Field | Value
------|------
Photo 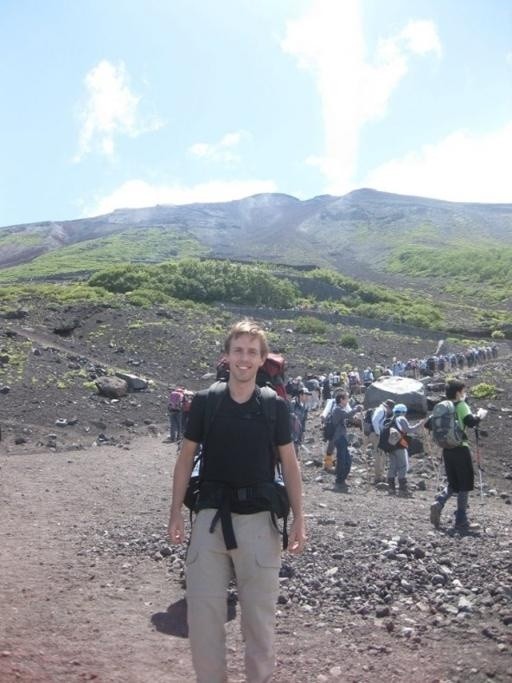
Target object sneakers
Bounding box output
[429,502,443,529]
[325,454,417,489]
[454,519,481,530]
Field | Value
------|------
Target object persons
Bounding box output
[365,399,426,491]
[322,387,358,484]
[167,387,194,442]
[289,387,312,439]
[168,321,306,682]
[428,378,482,531]
[284,343,498,411]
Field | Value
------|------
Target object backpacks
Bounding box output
[363,407,385,435]
[378,415,403,452]
[323,412,336,440]
[201,351,289,449]
[432,399,466,449]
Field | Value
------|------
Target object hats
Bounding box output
[297,388,313,396]
[382,399,407,413]
